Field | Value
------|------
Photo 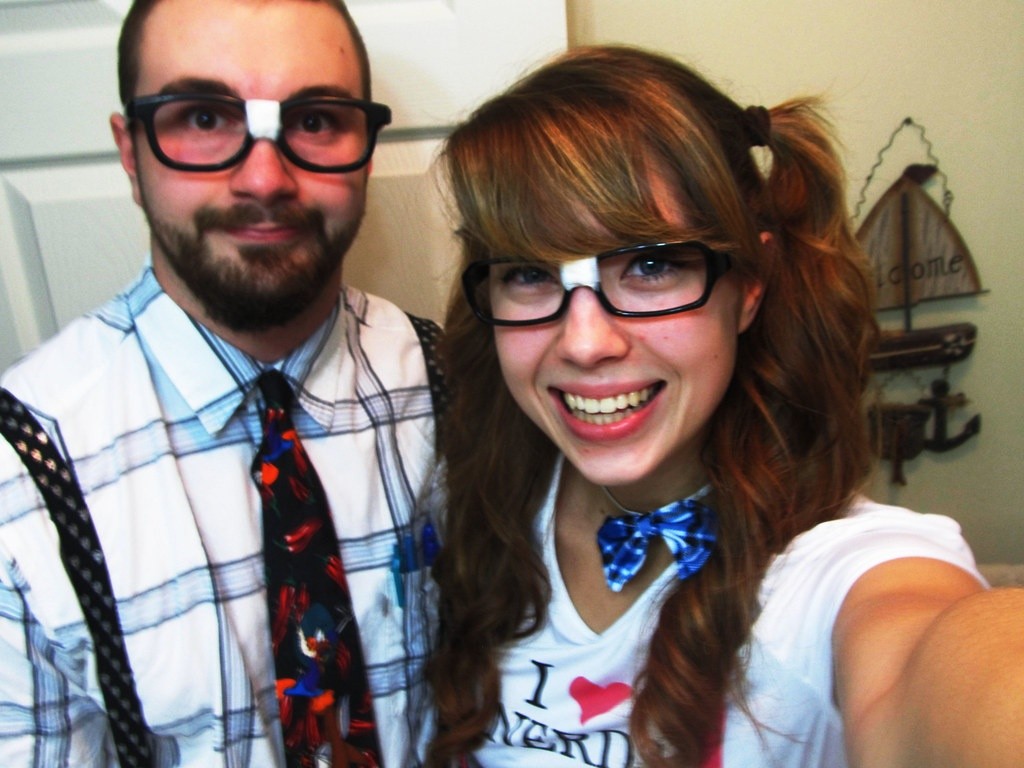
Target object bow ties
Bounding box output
[596,500,719,591]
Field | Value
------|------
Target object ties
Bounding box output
[250,372,383,768]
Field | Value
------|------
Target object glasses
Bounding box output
[127,92,391,174]
[461,241,734,327]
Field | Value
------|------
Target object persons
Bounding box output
[0,0,451,768]
[417,48,1024,768]
